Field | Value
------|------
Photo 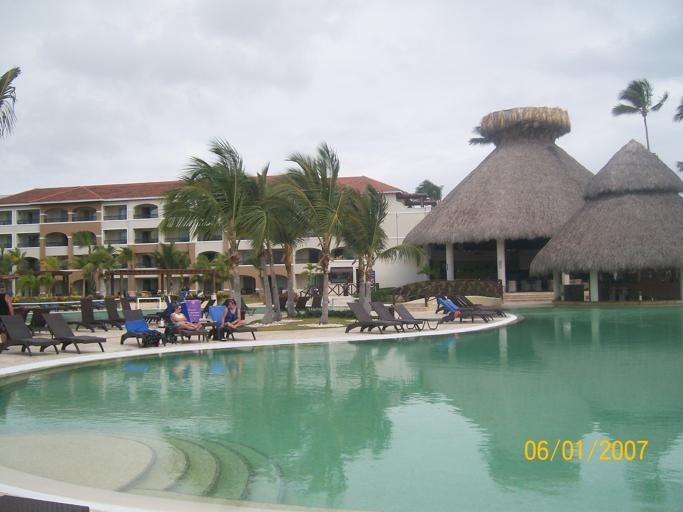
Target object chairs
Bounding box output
[271,293,323,315]
[344,301,443,334]
[0,293,107,357]
[436,294,506,324]
[68,293,260,349]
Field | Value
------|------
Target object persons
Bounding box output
[170,303,202,330]
[0,284,14,350]
[220,300,246,329]
